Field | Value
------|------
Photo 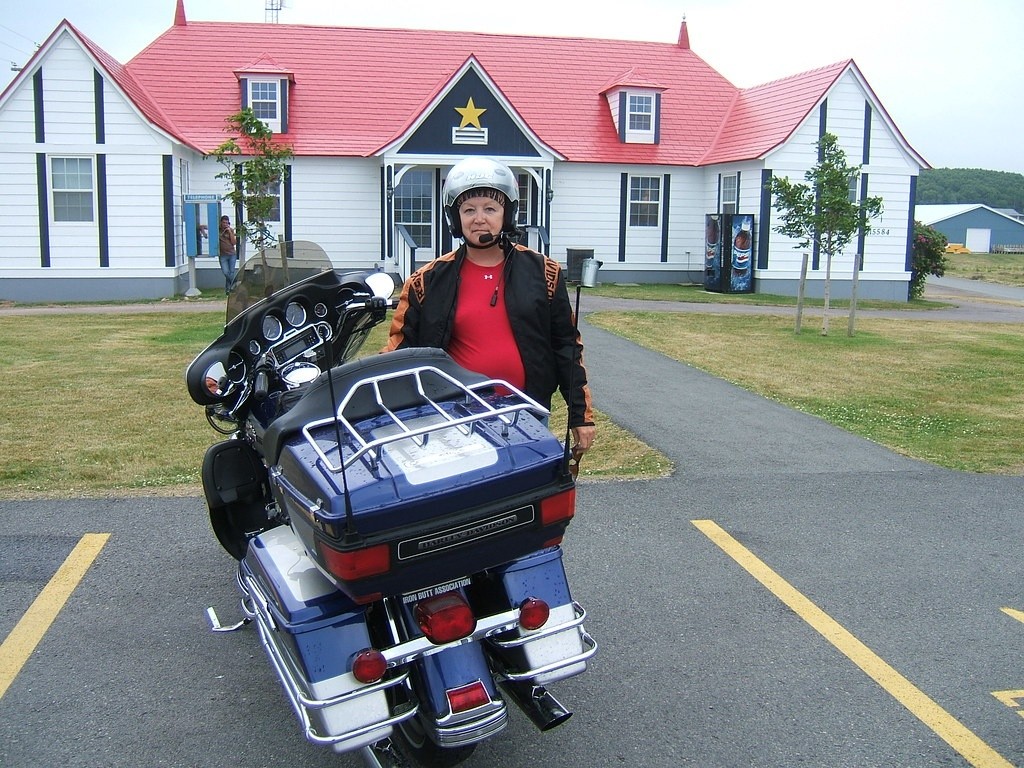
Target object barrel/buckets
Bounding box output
[566,248,595,282]
[384,261,394,273]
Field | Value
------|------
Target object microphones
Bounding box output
[479,231,515,243]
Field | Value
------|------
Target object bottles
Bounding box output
[374,262,378,272]
[705,215,720,269]
[732,221,752,276]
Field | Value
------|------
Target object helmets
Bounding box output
[442,156,526,239]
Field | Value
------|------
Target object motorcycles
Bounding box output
[186,242,598,768]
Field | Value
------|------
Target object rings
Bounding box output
[592,439,595,442]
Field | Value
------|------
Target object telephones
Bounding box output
[224,222,230,229]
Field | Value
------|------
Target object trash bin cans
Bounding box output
[581,257,603,289]
[566,248,595,283]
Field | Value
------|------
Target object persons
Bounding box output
[375,157,598,452]
[199,215,236,295]
